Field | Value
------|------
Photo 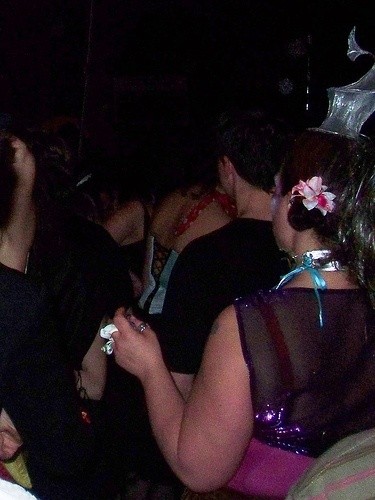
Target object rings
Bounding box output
[138,320,149,334]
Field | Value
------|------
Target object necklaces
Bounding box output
[282,248,347,275]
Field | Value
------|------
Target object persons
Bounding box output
[112,137,374,500]
[1,41,229,500]
[158,122,290,411]
